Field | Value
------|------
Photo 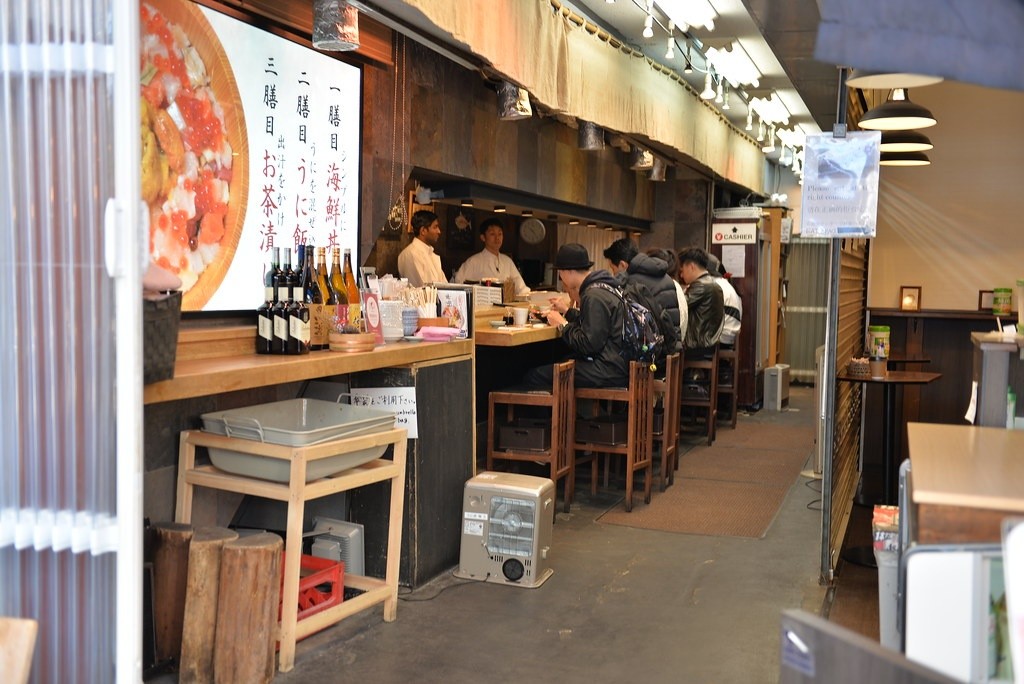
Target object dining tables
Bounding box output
[475,318,564,346]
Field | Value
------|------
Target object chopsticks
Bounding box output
[416,286,437,318]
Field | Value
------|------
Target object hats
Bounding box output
[706,254,723,277]
[548,243,595,269]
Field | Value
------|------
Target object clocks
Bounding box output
[519,219,546,245]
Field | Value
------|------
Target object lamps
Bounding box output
[845,67,944,88]
[606,0,803,186]
[881,130,934,152]
[880,152,931,166]
[858,88,938,130]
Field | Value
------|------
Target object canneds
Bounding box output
[993,287,1013,316]
[867,325,891,359]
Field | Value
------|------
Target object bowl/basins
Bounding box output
[403,308,418,336]
[490,321,506,327]
[533,312,564,323]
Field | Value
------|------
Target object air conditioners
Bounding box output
[453,471,554,589]
[764,364,791,413]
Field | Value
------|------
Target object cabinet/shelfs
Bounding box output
[712,202,793,416]
[780,421,1024,684]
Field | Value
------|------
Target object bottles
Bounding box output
[993,288,1012,315]
[870,326,890,356]
[256,244,361,354]
[502,307,514,326]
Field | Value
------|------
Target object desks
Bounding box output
[887,355,931,371]
[838,371,941,506]
[971,332,1024,429]
[144,324,477,593]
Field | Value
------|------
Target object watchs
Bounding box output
[557,321,568,333]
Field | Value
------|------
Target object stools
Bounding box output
[680,340,719,446]
[487,332,684,512]
[717,331,739,430]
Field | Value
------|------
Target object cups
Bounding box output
[418,318,449,330]
[513,308,528,325]
[869,361,887,377]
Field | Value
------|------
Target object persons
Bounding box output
[397,210,448,288]
[516,238,743,466]
[454,219,531,295]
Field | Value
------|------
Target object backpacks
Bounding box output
[585,282,665,372]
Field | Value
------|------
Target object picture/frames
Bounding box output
[898,285,922,312]
[978,290,994,311]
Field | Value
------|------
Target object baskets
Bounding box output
[144,289,182,387]
